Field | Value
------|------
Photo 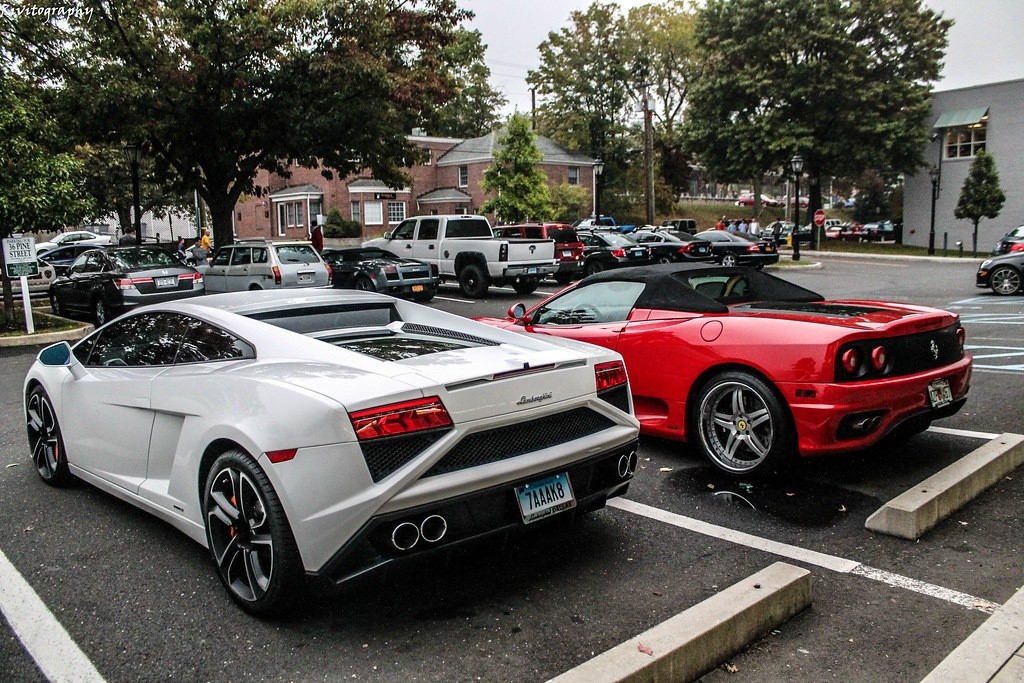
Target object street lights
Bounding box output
[927,163,942,254]
[125,138,150,245]
[592,155,610,229]
[791,150,805,259]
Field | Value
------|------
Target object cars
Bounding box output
[975,247,1024,297]
[691,228,781,274]
[624,224,712,267]
[573,228,653,277]
[991,224,1024,258]
[46,244,205,331]
[736,194,810,211]
[0,228,118,291]
[760,220,898,245]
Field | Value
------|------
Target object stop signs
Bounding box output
[813,210,828,228]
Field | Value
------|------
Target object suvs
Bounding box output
[489,220,587,287]
[189,238,333,299]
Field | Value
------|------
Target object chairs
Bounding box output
[694,281,728,300]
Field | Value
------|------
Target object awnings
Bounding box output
[933,106,988,128]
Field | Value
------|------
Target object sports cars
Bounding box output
[23,286,644,621]
[466,258,979,494]
[322,246,441,302]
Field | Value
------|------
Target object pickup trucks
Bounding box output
[357,214,560,297]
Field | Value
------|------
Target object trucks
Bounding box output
[574,216,640,234]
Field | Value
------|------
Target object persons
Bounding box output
[310,220,324,255]
[770,218,784,250]
[178,230,215,264]
[715,215,761,238]
[118,227,135,248]
